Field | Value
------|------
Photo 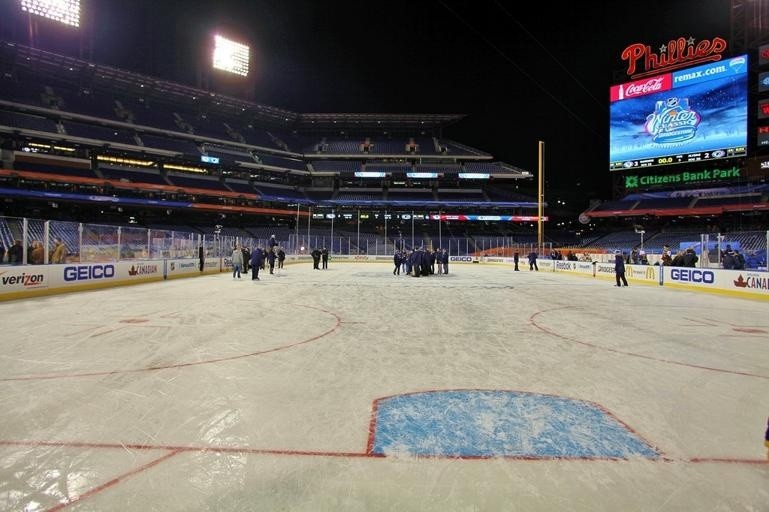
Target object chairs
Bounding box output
[552,190,769,273]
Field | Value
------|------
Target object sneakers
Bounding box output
[411,272,449,277]
[613,284,629,287]
[393,272,400,275]
[514,269,520,271]
[405,272,410,275]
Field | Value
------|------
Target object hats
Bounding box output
[613,250,621,254]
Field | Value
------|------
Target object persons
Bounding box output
[527,250,533,271]
[513,249,521,272]
[311,247,322,269]
[528,250,539,272]
[321,245,329,270]
[0,236,67,266]
[231,244,285,282]
[623,244,746,271]
[612,249,629,288]
[549,248,579,261]
[267,234,279,249]
[392,247,449,278]
